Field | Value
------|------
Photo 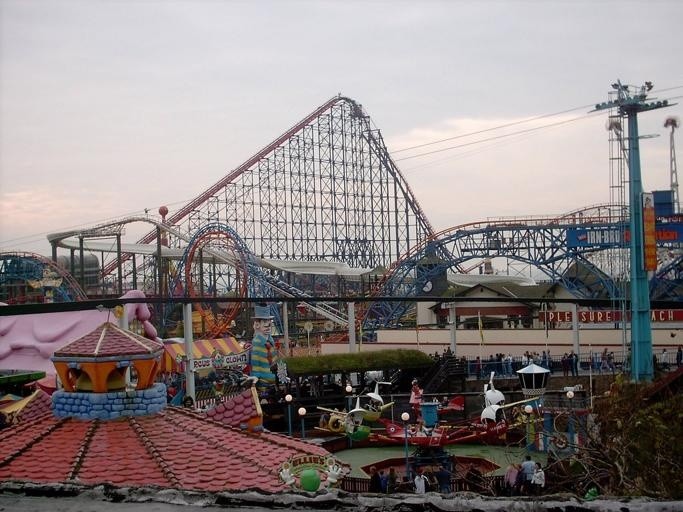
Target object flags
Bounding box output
[478,310,485,347]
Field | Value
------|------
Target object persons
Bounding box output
[514,455,545,493]
[429,347,466,369]
[415,467,451,494]
[653,347,683,371]
[432,396,451,407]
[600,347,631,371]
[476,353,513,379]
[513,405,527,422]
[562,350,579,375]
[522,349,554,374]
[370,399,380,412]
[240,306,279,393]
[371,466,398,494]
[466,464,481,493]
